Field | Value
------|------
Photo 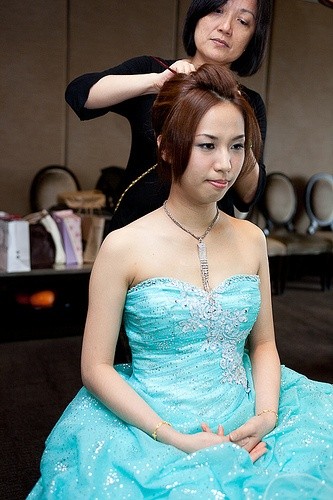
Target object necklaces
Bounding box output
[163,200,218,292]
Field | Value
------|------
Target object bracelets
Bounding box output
[151,421,171,440]
[255,409,278,423]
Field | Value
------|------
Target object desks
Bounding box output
[1,234,290,350]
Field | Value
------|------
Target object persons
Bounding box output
[24,63,332,500]
[64,0,269,234]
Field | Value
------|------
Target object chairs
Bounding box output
[305,172,333,291]
[250,205,289,295]
[258,172,329,295]
[30,165,81,213]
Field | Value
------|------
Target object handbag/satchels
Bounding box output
[0,190,105,273]
